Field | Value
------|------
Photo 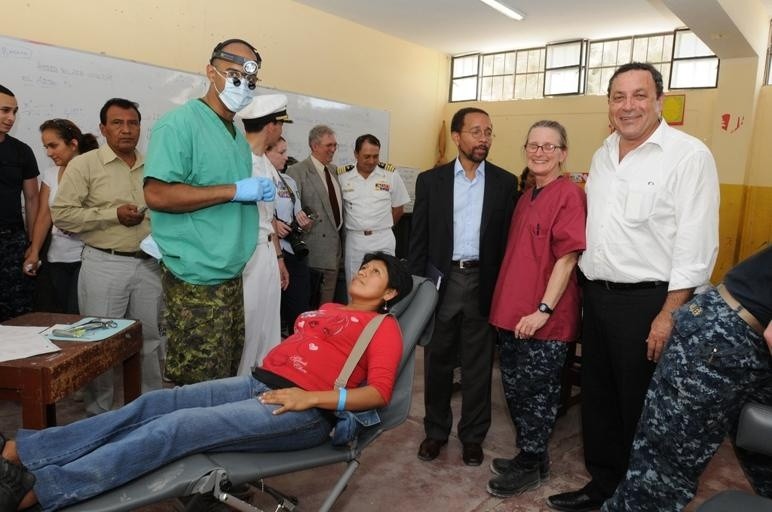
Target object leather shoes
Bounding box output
[461,442,484,468]
[545,477,620,512]
[417,433,449,462]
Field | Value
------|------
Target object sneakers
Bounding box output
[0,456,41,512]
[0,429,9,457]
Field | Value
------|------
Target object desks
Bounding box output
[2,312,144,431]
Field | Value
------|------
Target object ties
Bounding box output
[324,165,341,230]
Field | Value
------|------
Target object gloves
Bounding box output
[256,176,277,203]
[230,176,264,205]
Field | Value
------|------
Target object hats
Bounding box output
[235,93,296,126]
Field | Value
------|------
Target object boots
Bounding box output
[489,447,551,483]
[485,450,542,499]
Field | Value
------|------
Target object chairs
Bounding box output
[44,272,441,510]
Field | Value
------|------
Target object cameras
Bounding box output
[285,206,313,261]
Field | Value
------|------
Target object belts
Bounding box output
[586,278,670,293]
[346,227,392,236]
[449,259,480,269]
[86,241,155,261]
[715,282,765,337]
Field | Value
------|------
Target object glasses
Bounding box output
[523,141,565,154]
[456,129,496,139]
[211,62,261,86]
[316,142,340,150]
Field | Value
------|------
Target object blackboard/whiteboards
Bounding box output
[0,35,393,214]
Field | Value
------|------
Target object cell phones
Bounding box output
[139,207,148,213]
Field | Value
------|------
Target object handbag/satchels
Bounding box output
[329,409,382,446]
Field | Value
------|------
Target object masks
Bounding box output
[212,65,257,113]
